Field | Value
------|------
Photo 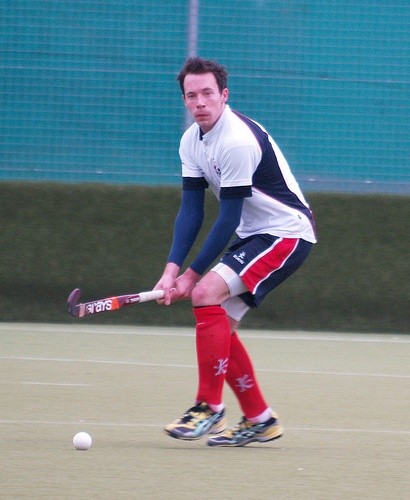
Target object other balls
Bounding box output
[73,432,92,450]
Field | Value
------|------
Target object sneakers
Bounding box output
[164,401,226,441]
[206,410,284,448]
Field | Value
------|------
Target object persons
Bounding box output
[154,56,318,447]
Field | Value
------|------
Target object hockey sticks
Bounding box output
[67,287,175,319]
